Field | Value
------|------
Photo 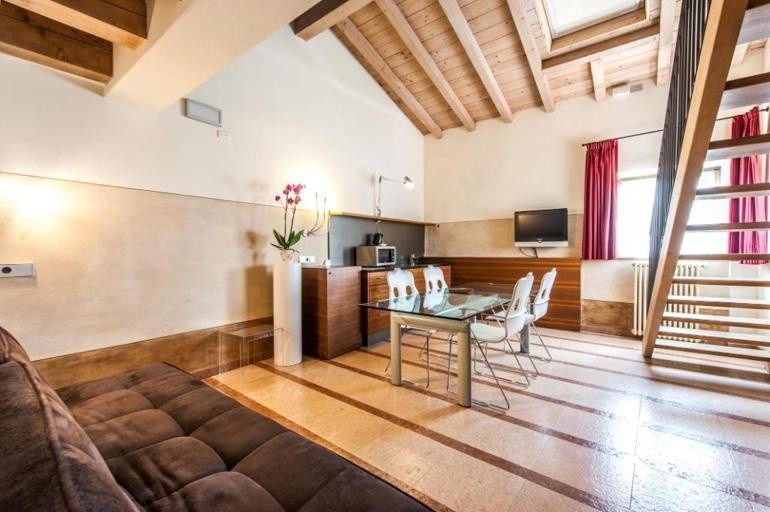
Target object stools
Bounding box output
[217,319,287,394]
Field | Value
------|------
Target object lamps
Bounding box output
[376,174,414,223]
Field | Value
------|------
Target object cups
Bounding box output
[322,260,331,267]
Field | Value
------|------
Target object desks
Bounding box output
[358,280,541,407]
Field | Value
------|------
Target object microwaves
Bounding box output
[354,246,397,268]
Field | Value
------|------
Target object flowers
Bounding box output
[269,183,306,250]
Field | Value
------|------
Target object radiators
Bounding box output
[629,261,706,343]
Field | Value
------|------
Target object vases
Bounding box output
[272,252,304,367]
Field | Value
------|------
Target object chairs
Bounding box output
[385,265,558,409]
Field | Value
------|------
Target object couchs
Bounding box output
[0,326,434,512]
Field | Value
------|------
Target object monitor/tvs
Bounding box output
[514,208,569,248]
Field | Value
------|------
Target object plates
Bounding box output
[449,287,475,294]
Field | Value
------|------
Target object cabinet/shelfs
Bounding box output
[302,263,363,360]
[361,264,452,347]
